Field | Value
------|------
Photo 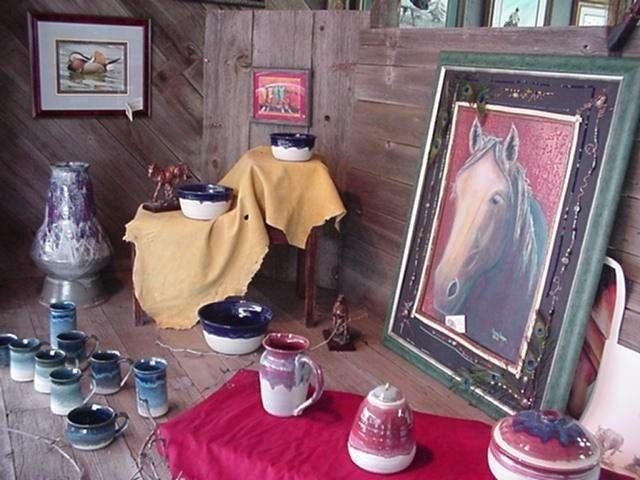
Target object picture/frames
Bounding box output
[250,67,314,129]
[378,48,638,423]
[26,9,153,119]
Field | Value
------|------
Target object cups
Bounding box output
[0,301,169,451]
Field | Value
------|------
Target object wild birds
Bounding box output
[66,50,121,75]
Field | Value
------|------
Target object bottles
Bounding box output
[347,383,417,474]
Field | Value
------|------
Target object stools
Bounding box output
[131,145,324,330]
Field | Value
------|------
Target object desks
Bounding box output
[168,368,637,480]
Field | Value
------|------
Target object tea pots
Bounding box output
[259,332,325,418]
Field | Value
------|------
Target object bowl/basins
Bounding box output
[198,301,275,356]
[178,182,234,220]
[269,132,316,162]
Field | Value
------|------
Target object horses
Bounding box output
[432,115,550,363]
